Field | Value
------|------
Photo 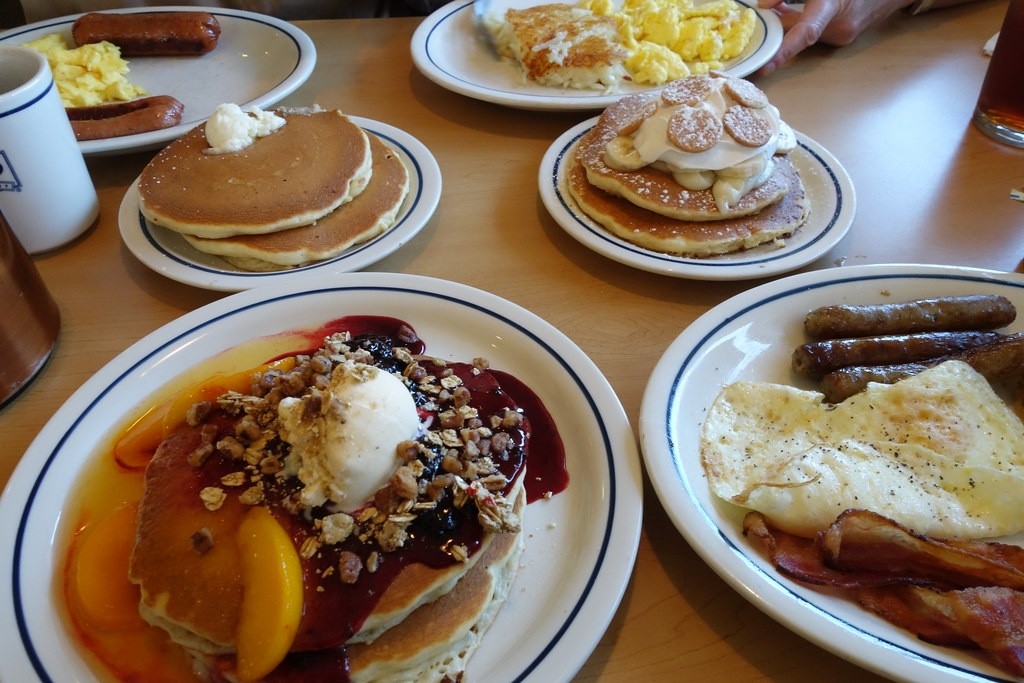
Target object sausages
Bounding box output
[63,95,183,138]
[72,11,221,57]
[793,292,1022,404]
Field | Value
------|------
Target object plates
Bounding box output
[536,112,858,283]
[115,110,442,294]
[639,264,1023,682]
[0,4,316,159]
[1,272,647,682]
[410,0,785,110]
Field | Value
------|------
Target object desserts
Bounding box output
[569,73,808,251]
[132,331,523,682]
[136,103,408,272]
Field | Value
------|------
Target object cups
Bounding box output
[0,46,100,255]
[2,212,65,411]
[970,1,1024,148]
